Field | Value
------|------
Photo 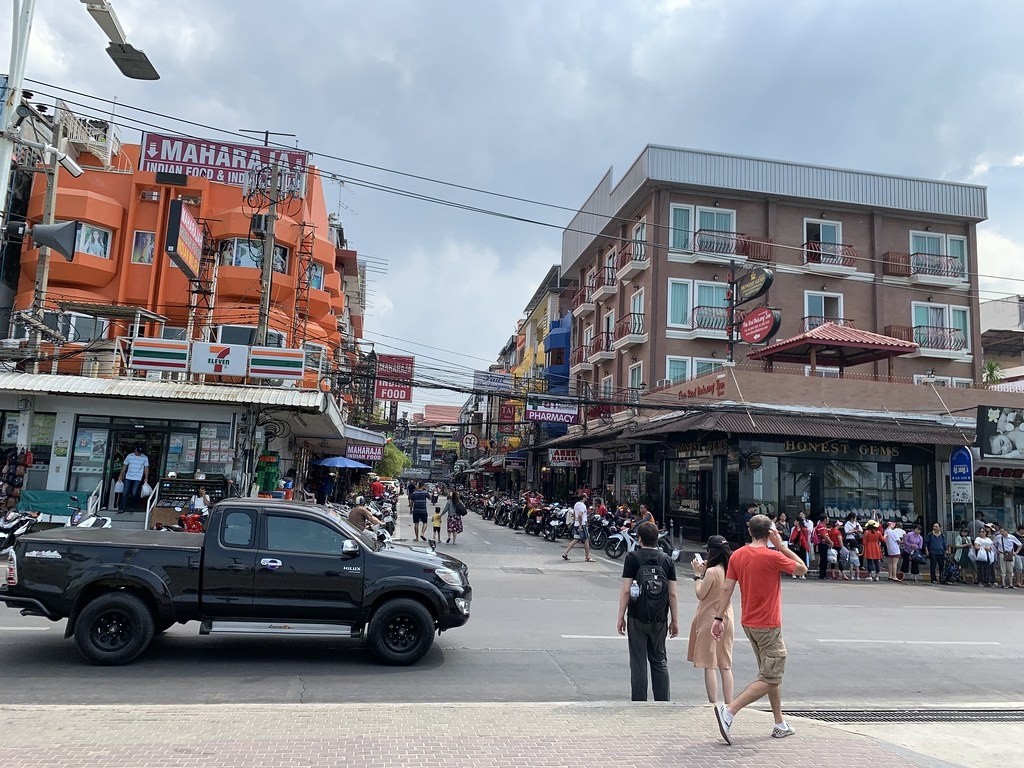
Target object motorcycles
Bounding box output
[432,490,439,505]
[0,505,41,552]
[153,506,208,534]
[322,486,398,550]
[457,486,673,559]
[64,496,113,529]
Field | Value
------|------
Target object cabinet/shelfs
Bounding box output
[157,478,225,506]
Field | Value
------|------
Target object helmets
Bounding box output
[355,495,365,505]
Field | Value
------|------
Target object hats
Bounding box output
[865,520,880,528]
[136,445,142,453]
[984,523,997,531]
[579,493,590,498]
[702,535,729,550]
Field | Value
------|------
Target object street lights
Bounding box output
[0,1,161,226]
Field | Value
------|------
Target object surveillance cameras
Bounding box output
[57,152,84,178]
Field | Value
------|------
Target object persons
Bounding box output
[688,535,734,703]
[617,521,680,701]
[311,263,320,290]
[767,510,1024,589]
[561,493,596,562]
[743,504,758,545]
[452,484,496,504]
[980,408,1024,459]
[431,507,442,543]
[191,486,210,525]
[710,515,808,746]
[438,492,465,544]
[117,446,149,514]
[321,472,385,502]
[410,482,431,541]
[349,496,386,540]
[222,241,285,272]
[83,229,104,257]
[401,480,451,506]
[595,498,655,528]
[519,489,543,528]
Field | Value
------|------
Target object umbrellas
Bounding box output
[309,455,373,470]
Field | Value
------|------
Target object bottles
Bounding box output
[7,555,16,585]
[630,580,639,602]
[58,437,67,447]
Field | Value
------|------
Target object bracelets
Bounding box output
[693,576,702,581]
[714,616,723,621]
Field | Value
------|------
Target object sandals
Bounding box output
[561,552,596,562]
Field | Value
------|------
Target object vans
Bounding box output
[379,479,401,495]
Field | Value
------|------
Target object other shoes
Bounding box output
[118,509,123,513]
[790,572,1024,590]
[413,534,457,545]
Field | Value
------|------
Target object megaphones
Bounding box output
[7,218,78,262]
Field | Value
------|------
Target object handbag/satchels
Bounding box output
[909,548,928,566]
[456,501,467,516]
[114,479,152,498]
[828,547,838,564]
[0,447,33,508]
[968,544,1013,561]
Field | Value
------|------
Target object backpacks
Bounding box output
[631,549,669,625]
[780,522,790,541]
[812,527,826,545]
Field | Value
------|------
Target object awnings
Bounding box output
[463,455,503,473]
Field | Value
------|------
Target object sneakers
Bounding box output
[714,704,795,745]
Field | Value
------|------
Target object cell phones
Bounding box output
[695,553,703,566]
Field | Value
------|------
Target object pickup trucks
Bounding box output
[1,497,472,667]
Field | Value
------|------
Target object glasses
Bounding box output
[753,508,756,511]
[979,530,985,533]
[200,487,205,490]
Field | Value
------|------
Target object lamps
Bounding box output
[631,356,637,364]
[604,306,611,311]
[640,380,647,389]
[927,294,933,302]
[715,200,720,207]
[821,212,826,218]
[821,283,828,290]
[587,264,593,271]
[928,367,937,379]
[633,284,640,291]
[714,273,719,281]
[711,349,717,358]
[586,320,591,326]
[635,214,642,222]
[604,369,609,375]
[608,242,613,250]
